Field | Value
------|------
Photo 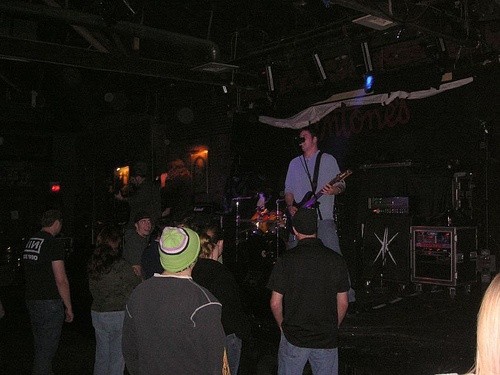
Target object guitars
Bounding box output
[287,168,353,235]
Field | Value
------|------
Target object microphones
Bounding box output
[294,136,305,143]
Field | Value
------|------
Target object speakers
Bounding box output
[361,214,423,285]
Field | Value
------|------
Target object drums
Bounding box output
[232,196,257,219]
[252,205,287,233]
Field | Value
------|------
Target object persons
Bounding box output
[23,166,349,375]
[284,126,355,302]
[461,273,500,375]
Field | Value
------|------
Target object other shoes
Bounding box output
[345,302,357,319]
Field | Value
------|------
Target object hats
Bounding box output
[158,226,200,272]
[294,208,317,233]
[134,212,151,223]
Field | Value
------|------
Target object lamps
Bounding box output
[311,54,327,82]
[359,42,376,93]
[435,37,448,57]
[265,65,279,97]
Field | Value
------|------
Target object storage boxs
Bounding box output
[408,226,479,287]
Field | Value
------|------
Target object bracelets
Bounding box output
[337,187,343,194]
